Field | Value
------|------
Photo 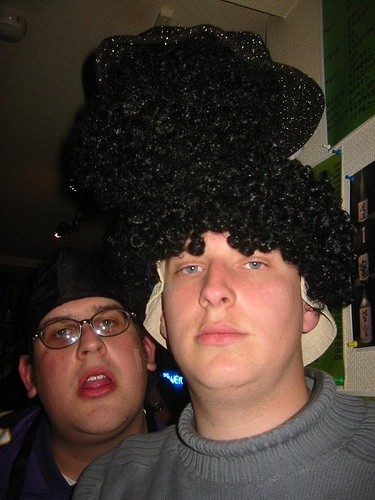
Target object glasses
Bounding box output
[30,307,142,350]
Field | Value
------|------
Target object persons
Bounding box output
[72,159,375,499]
[145,377,184,429]
[0,255,182,500]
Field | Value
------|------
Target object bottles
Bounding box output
[359,286,372,344]
[358,226,370,283]
[357,173,369,223]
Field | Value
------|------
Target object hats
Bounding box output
[24,246,129,355]
